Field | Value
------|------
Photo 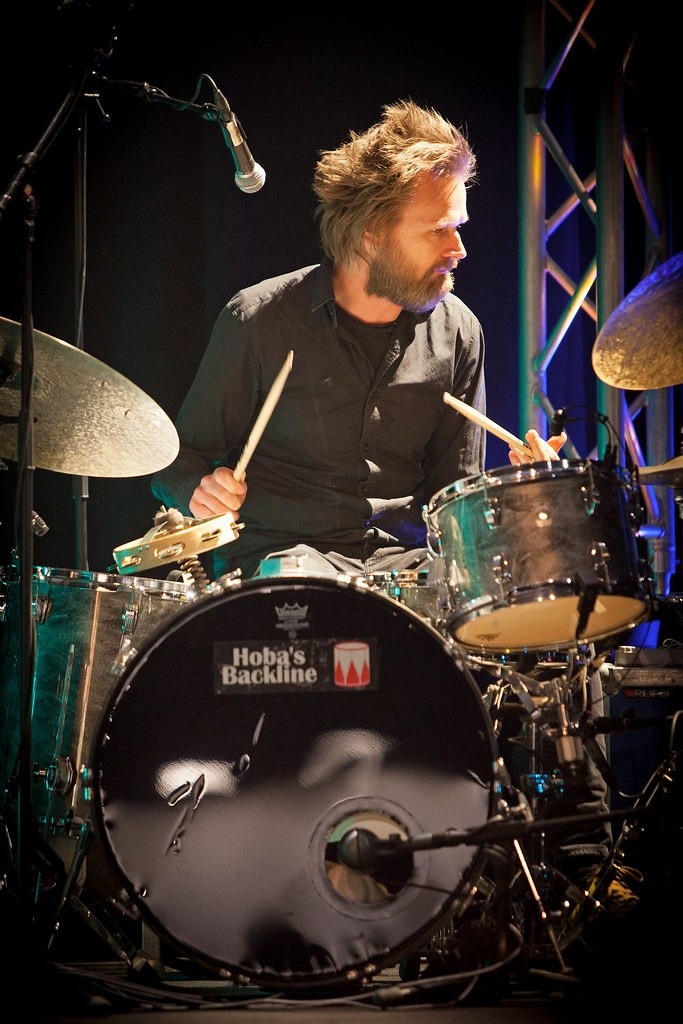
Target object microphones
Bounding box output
[213,89,266,193]
[626,715,674,730]
[549,409,566,440]
[337,828,476,871]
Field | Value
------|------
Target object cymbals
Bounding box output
[156,542,185,560]
[107,556,141,572]
[632,455,683,486]
[0,314,181,479]
[230,522,245,531]
[200,532,222,544]
[590,252,683,391]
[104,510,247,576]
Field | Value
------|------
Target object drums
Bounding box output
[30,564,224,838]
[87,576,498,985]
[427,458,651,653]
[338,571,442,631]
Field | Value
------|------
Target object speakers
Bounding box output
[590,665,683,901]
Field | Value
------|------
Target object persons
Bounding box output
[151,103,663,950]
